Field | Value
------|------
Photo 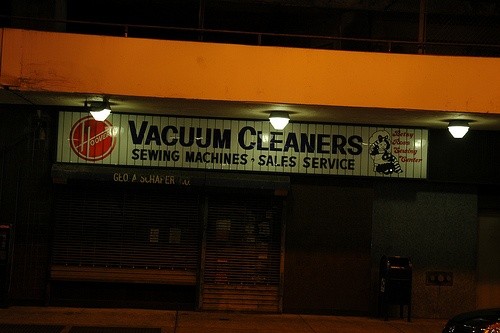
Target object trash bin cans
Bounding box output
[376,253,411,321]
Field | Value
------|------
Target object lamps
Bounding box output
[269,112,290,130]
[88,100,113,121]
[447,120,470,138]
